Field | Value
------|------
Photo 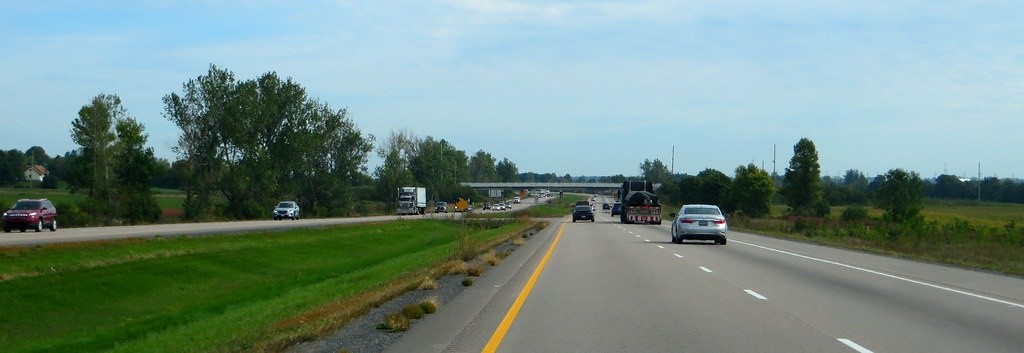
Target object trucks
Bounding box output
[396,186,426,215]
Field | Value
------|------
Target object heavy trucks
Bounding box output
[617,177,662,225]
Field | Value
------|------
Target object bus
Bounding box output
[456,197,474,211]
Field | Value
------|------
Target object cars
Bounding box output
[571,207,595,222]
[603,203,611,210]
[504,201,511,209]
[434,202,448,213]
[483,202,491,210]
[669,204,728,246]
[594,194,598,197]
[3,197,57,232]
[572,200,594,210]
[273,201,300,221]
[499,203,505,210]
[591,197,596,201]
[524,190,554,200]
[590,203,596,211]
[492,204,500,211]
[513,197,520,204]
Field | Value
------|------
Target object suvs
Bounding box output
[611,202,621,217]
